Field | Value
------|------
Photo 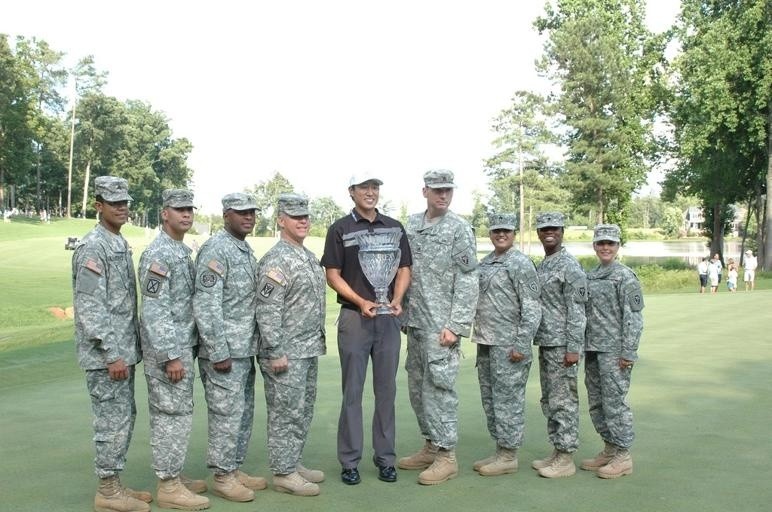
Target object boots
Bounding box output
[157,476,210,511]
[472,448,498,471]
[114,475,153,504]
[232,469,267,490]
[532,450,561,469]
[213,471,255,502]
[156,474,208,493]
[539,452,576,479]
[480,447,517,475]
[596,449,633,479]
[399,439,440,470]
[95,476,151,512]
[296,463,325,483]
[418,451,458,485]
[581,443,618,471]
[273,471,320,496]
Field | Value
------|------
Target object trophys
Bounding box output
[356,233,402,316]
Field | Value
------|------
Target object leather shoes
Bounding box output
[342,467,361,484]
[378,465,398,482]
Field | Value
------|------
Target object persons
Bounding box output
[74,175,153,512]
[139,190,212,511]
[192,192,267,503]
[726,265,738,294]
[533,210,589,481]
[698,258,708,294]
[254,193,328,498]
[397,170,479,487]
[727,258,738,292]
[708,258,719,294]
[742,250,758,292]
[712,254,722,293]
[584,224,644,481]
[322,166,411,485]
[474,212,541,477]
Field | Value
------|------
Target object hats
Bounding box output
[278,195,312,216]
[348,168,384,188]
[710,259,716,263]
[95,176,134,202]
[162,189,197,210]
[745,250,752,254]
[423,168,458,189]
[593,224,620,243]
[535,213,565,229]
[489,214,517,231]
[221,193,262,212]
